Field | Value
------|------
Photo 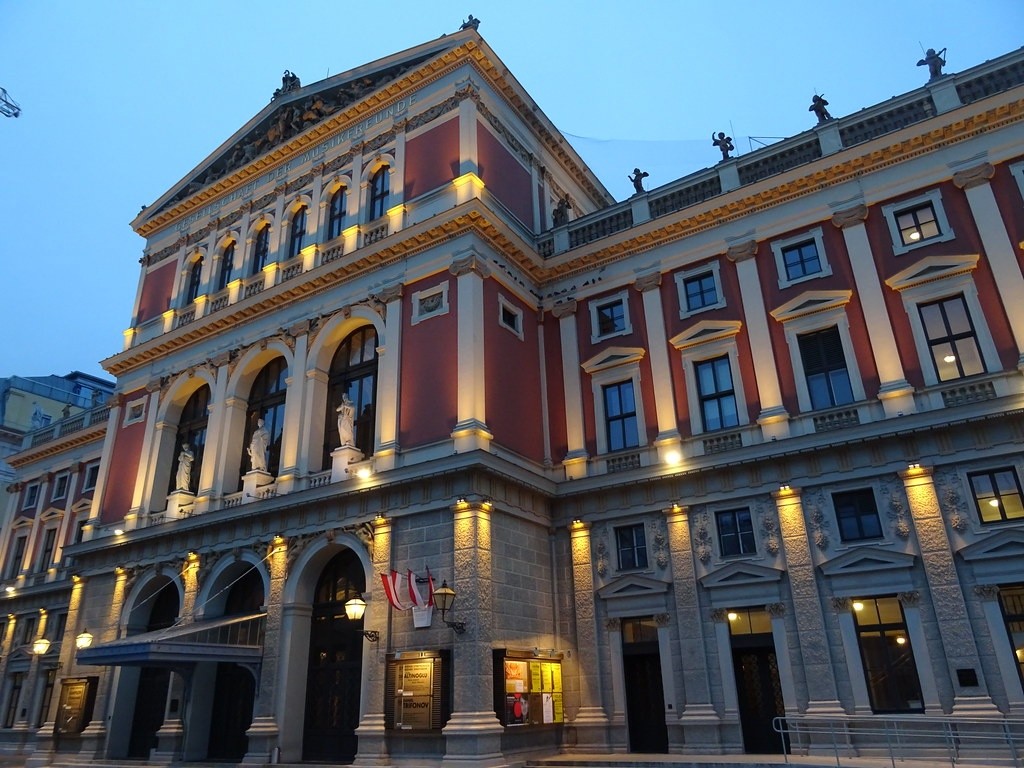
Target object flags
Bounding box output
[381,570,435,611]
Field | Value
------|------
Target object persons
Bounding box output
[553,198,572,225]
[280,70,301,94]
[809,95,833,122]
[628,168,649,193]
[337,393,355,447]
[176,443,194,491]
[460,14,481,31]
[925,48,948,76]
[711,132,734,159]
[61,401,73,418]
[91,388,103,406]
[247,418,269,471]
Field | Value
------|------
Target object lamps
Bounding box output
[76,628,94,647]
[33,634,51,654]
[344,579,466,642]
